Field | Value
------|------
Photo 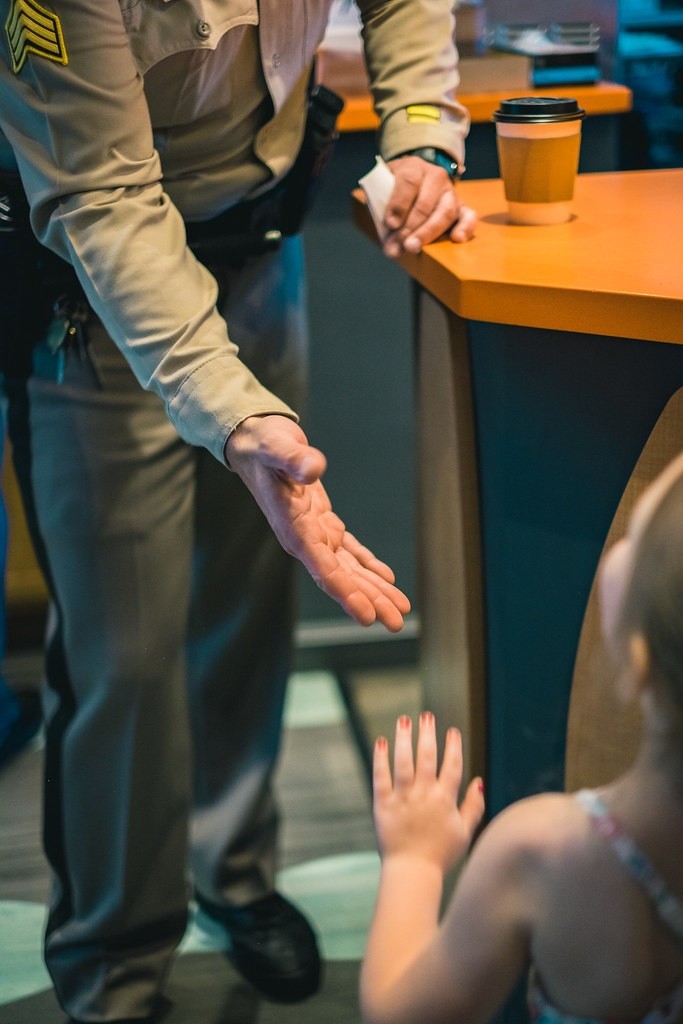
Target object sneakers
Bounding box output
[192,887,321,1002]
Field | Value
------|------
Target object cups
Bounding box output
[490,96,586,226]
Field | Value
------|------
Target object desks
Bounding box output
[348,163,683,1024]
[274,52,629,676]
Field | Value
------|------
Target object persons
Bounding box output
[358,455,682,1024]
[0,0,477,1022]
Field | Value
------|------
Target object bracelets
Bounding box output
[411,147,457,179]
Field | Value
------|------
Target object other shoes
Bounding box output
[0,688,43,762]
